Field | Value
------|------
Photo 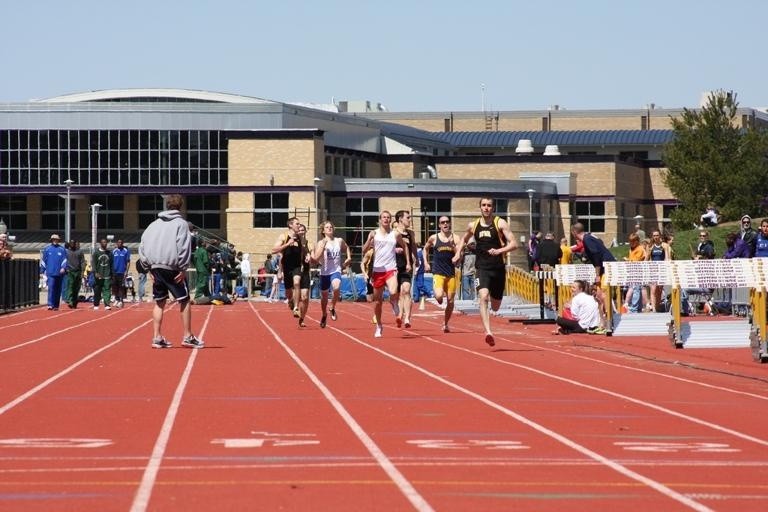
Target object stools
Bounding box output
[687,293,720,319]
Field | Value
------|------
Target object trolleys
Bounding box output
[682,287,723,318]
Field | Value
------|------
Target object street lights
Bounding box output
[0,218,9,235]
[63,178,75,242]
[268,174,276,228]
[311,175,323,244]
[632,213,643,228]
[525,188,537,236]
[90,202,102,252]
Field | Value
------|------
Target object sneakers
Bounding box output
[371,296,449,338]
[90,300,123,310]
[151,336,174,348]
[181,336,204,349]
[485,333,495,347]
[288,299,337,328]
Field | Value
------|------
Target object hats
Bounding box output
[49,234,61,241]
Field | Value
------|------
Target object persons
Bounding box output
[736,213,759,257]
[526,205,718,335]
[0,230,14,260]
[184,235,252,304]
[137,194,207,350]
[360,210,425,337]
[42,234,131,311]
[752,219,768,259]
[135,258,148,303]
[422,216,464,331]
[721,232,752,258]
[451,196,521,347]
[259,218,352,328]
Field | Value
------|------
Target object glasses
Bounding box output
[742,222,750,224]
[699,235,705,237]
